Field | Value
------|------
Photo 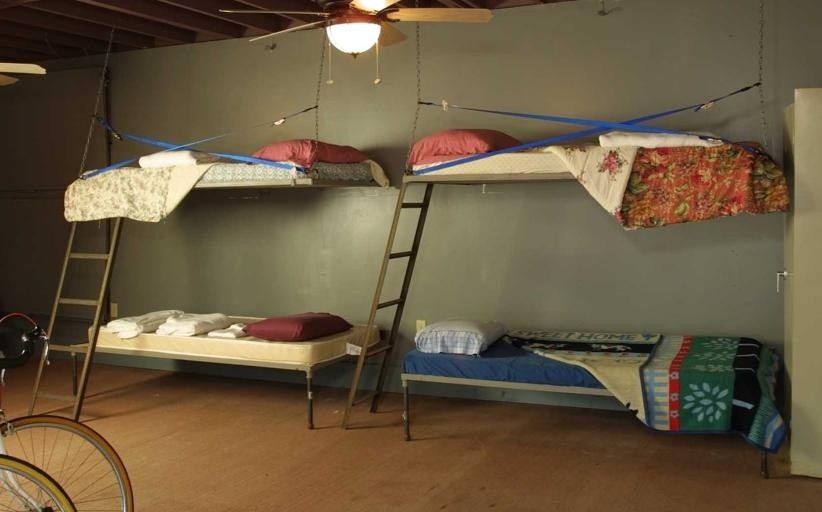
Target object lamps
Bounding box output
[323,16,381,86]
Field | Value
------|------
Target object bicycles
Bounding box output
[0,312,135,512]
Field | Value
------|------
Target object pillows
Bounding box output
[249,136,369,168]
[403,127,527,170]
[413,317,508,354]
[241,312,354,342]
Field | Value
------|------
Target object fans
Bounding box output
[217,1,496,42]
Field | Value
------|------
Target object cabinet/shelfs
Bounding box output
[775,86,822,480]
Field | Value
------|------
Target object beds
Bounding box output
[405,144,780,187]
[86,311,388,430]
[64,157,393,224]
[400,328,782,479]
